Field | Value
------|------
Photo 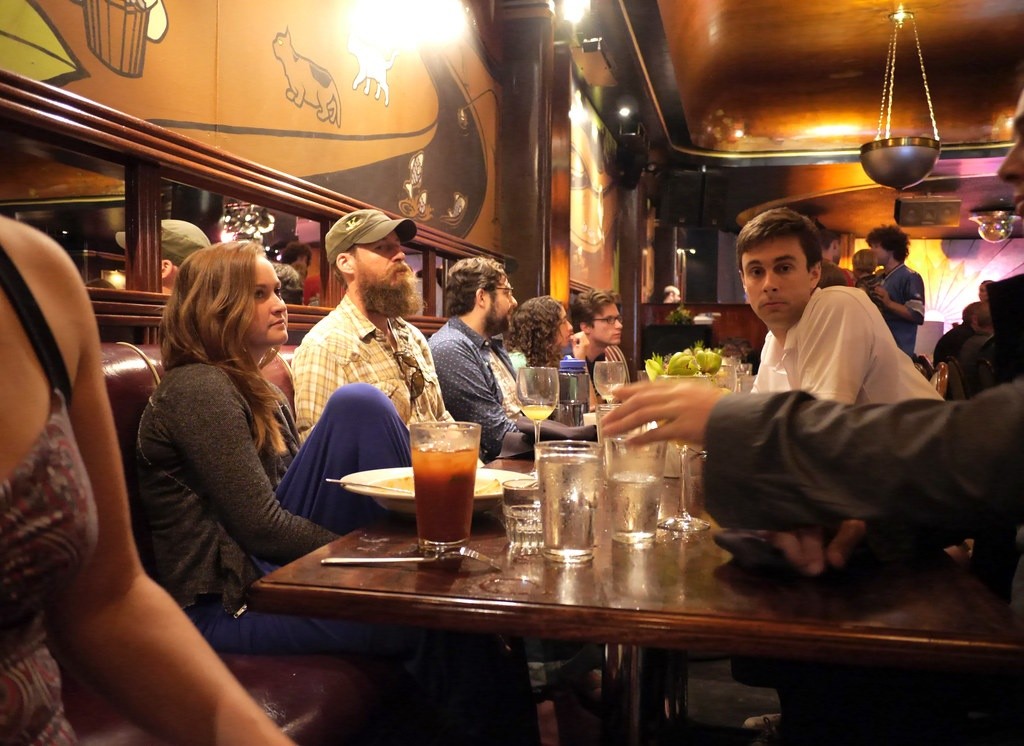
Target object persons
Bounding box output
[601,66,1024,746]
[115,218,213,294]
[131,211,635,745]
[0,213,296,746]
[815,224,925,361]
[735,205,980,746]
[932,280,994,392]
[266,261,304,306]
[662,285,681,303]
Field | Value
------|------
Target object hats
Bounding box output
[116,220,211,267]
[325,210,416,264]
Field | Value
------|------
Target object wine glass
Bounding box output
[656,417,711,533]
[515,365,560,477]
[593,361,626,404]
[714,364,738,393]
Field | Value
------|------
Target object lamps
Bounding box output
[969,211,1021,243]
[859,2,941,194]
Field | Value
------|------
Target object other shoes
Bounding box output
[741,712,782,746]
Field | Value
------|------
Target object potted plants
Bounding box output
[640,299,714,369]
[644,340,731,449]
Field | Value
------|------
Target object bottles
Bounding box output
[558,355,590,427]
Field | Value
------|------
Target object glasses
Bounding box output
[497,287,513,295]
[394,351,425,400]
[593,316,623,323]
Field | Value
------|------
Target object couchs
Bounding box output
[100,343,391,746]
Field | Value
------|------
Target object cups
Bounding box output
[595,403,640,442]
[604,434,667,545]
[535,439,603,566]
[501,478,544,550]
[409,421,482,558]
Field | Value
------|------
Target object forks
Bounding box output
[321,545,502,570]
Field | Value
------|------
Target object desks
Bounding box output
[246,459,1024,746]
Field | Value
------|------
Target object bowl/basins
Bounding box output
[341,467,533,516]
